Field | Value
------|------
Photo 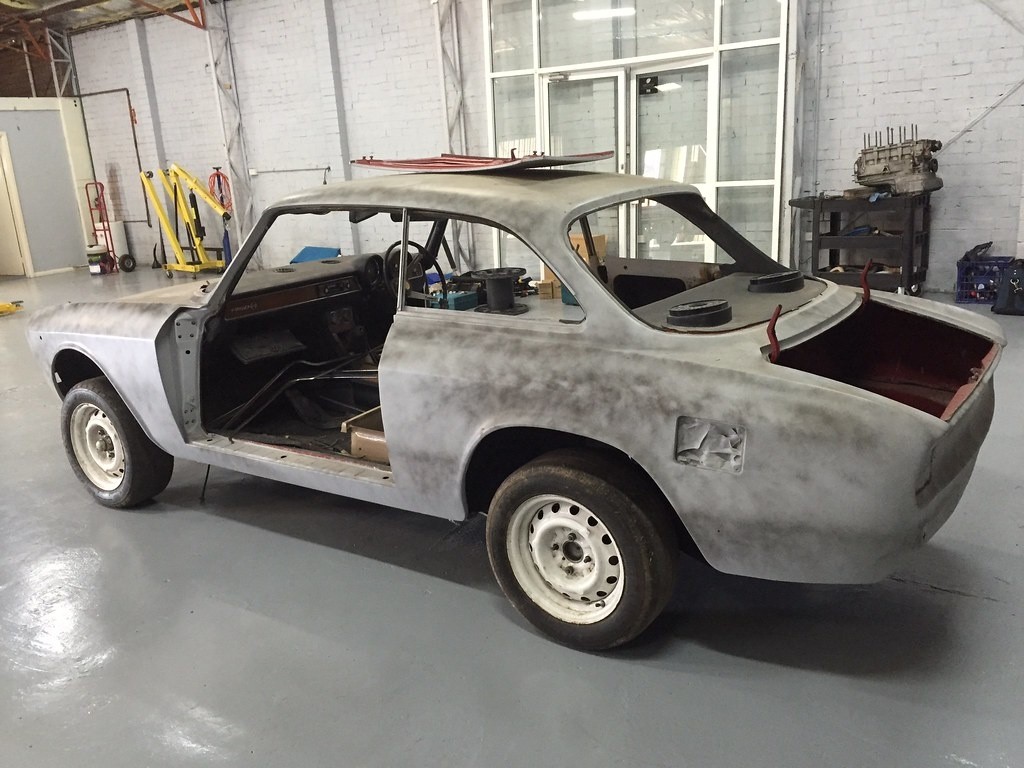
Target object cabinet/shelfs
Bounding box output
[788,190,931,297]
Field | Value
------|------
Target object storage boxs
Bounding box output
[536,234,607,299]
[955,256,1015,304]
[433,288,478,311]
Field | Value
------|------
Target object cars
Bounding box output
[28,168,1010,655]
